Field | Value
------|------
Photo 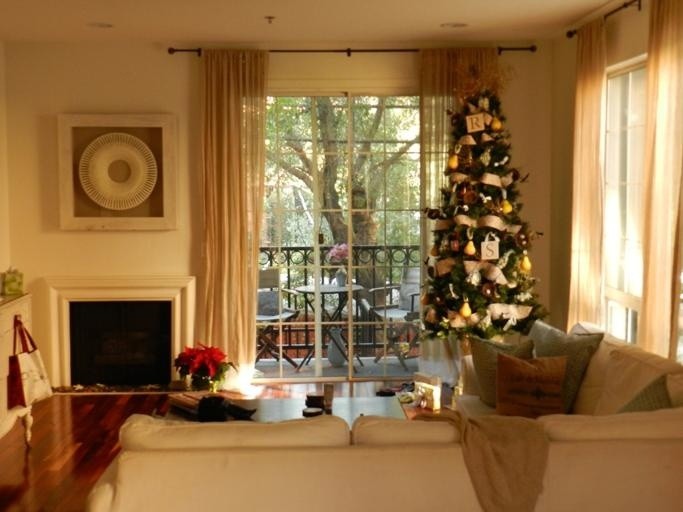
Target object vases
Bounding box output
[335,265,348,286]
[326,327,346,367]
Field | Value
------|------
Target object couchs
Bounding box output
[85,407,484,512]
[456,318,683,512]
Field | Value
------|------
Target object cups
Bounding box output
[323,383,332,410]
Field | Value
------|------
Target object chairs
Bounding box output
[256,267,299,368]
[368,266,421,370]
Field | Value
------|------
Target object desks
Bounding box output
[294,283,365,374]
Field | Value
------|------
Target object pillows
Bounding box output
[527,319,605,415]
[469,334,537,408]
[494,352,569,415]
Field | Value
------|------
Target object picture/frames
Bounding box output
[57,112,176,232]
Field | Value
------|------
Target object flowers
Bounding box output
[171,341,239,392]
[326,242,349,264]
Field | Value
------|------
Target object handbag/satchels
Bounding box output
[7,349,53,411]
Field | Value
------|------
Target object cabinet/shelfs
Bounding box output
[0,289,35,445]
